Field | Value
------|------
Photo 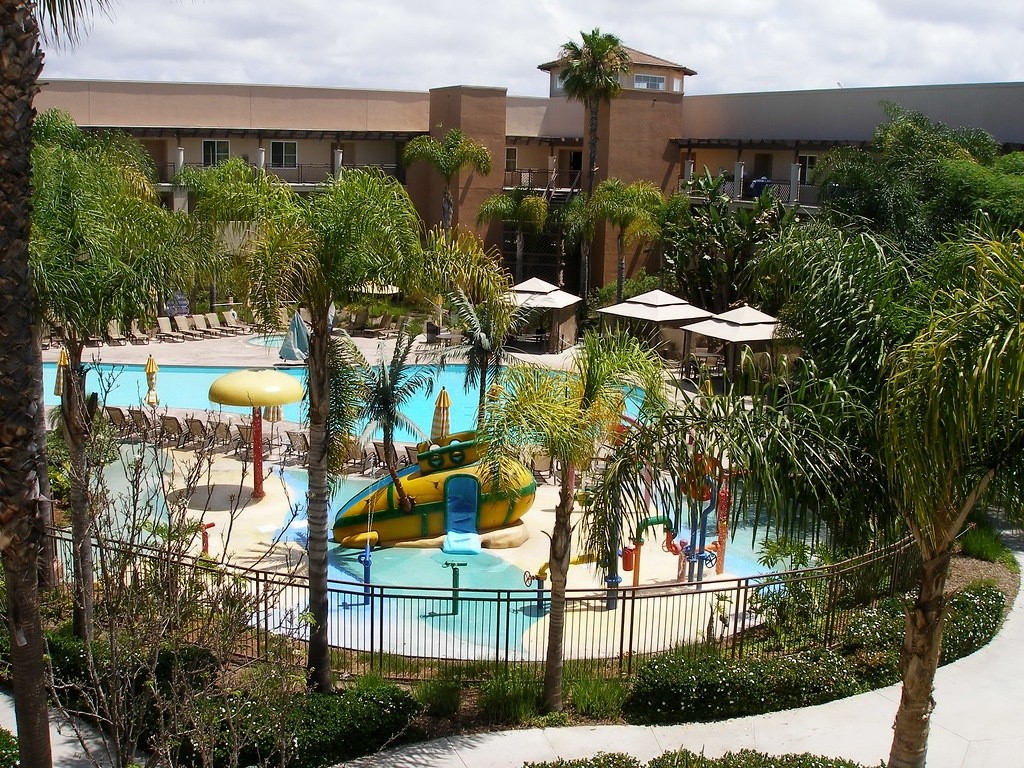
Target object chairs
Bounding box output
[695,347,708,362]
[41,308,409,350]
[104,406,422,476]
[523,449,554,479]
[705,356,720,372]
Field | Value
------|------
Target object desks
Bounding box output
[502,334,546,355]
[690,353,721,379]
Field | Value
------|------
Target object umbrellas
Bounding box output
[341,277,402,329]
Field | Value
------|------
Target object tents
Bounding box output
[482,277,583,354]
[593,288,805,385]
